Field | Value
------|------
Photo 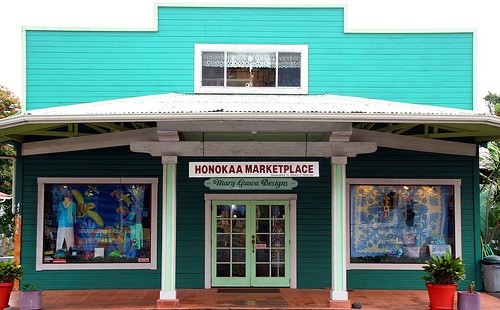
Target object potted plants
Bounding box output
[0,258,24,310]
[421,250,466,310]
